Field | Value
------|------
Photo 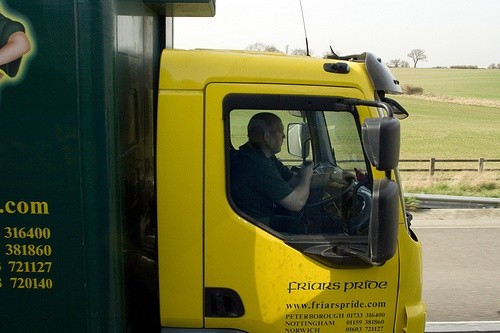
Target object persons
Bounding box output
[230,112,315,234]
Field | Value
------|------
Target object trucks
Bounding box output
[0,0,429,333]
